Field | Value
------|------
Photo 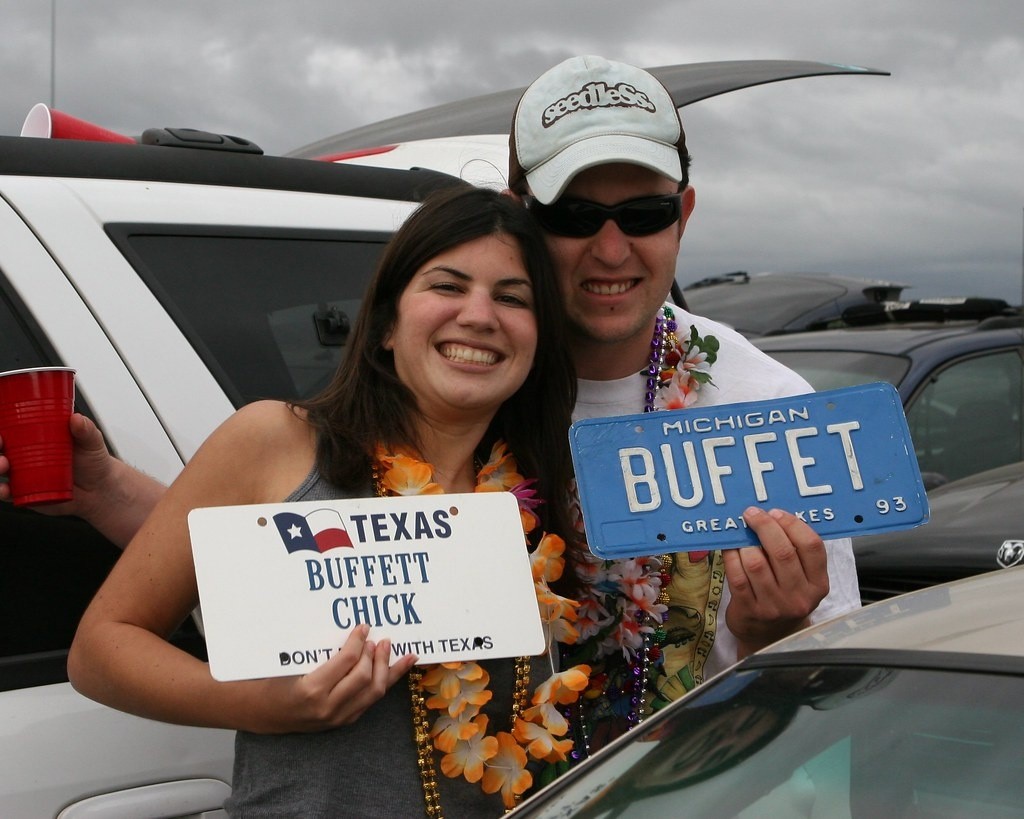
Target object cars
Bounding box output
[501,564,1024,818]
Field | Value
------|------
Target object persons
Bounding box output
[66,189,580,819]
[0,55,861,819]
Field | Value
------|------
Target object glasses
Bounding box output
[518,188,683,237]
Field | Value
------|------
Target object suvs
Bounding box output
[0,57,890,818]
[680,266,1022,599]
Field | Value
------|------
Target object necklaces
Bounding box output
[364,302,719,819]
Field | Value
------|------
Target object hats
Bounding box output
[507,55,688,205]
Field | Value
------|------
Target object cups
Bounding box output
[0,367,78,506]
[20,103,139,145]
